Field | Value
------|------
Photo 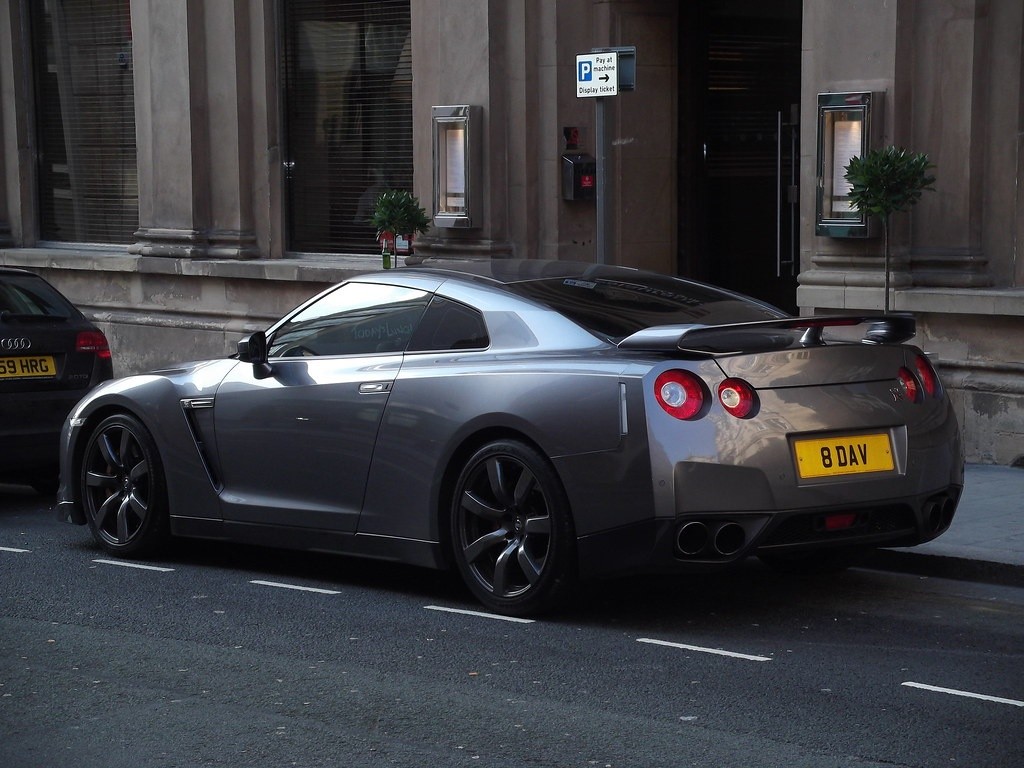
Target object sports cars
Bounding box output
[58,258,968,615]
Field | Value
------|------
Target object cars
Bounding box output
[0,266,114,496]
